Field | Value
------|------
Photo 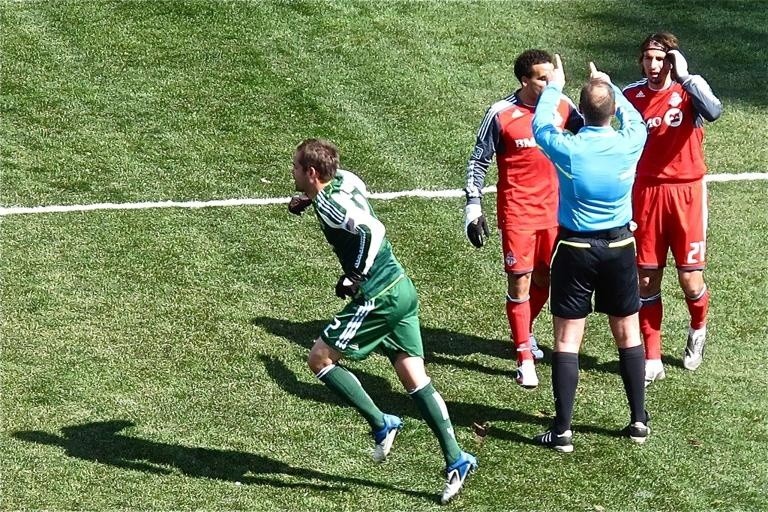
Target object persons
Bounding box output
[284,136,479,505]
[621,30,724,390]
[531,53,651,456]
[462,49,586,391]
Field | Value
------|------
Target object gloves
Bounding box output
[464,195,492,248]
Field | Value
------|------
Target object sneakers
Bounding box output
[510,327,544,360]
[644,359,665,388]
[630,409,651,442]
[516,348,539,387]
[440,451,476,503]
[532,429,573,453]
[373,413,401,463]
[683,321,706,371]
